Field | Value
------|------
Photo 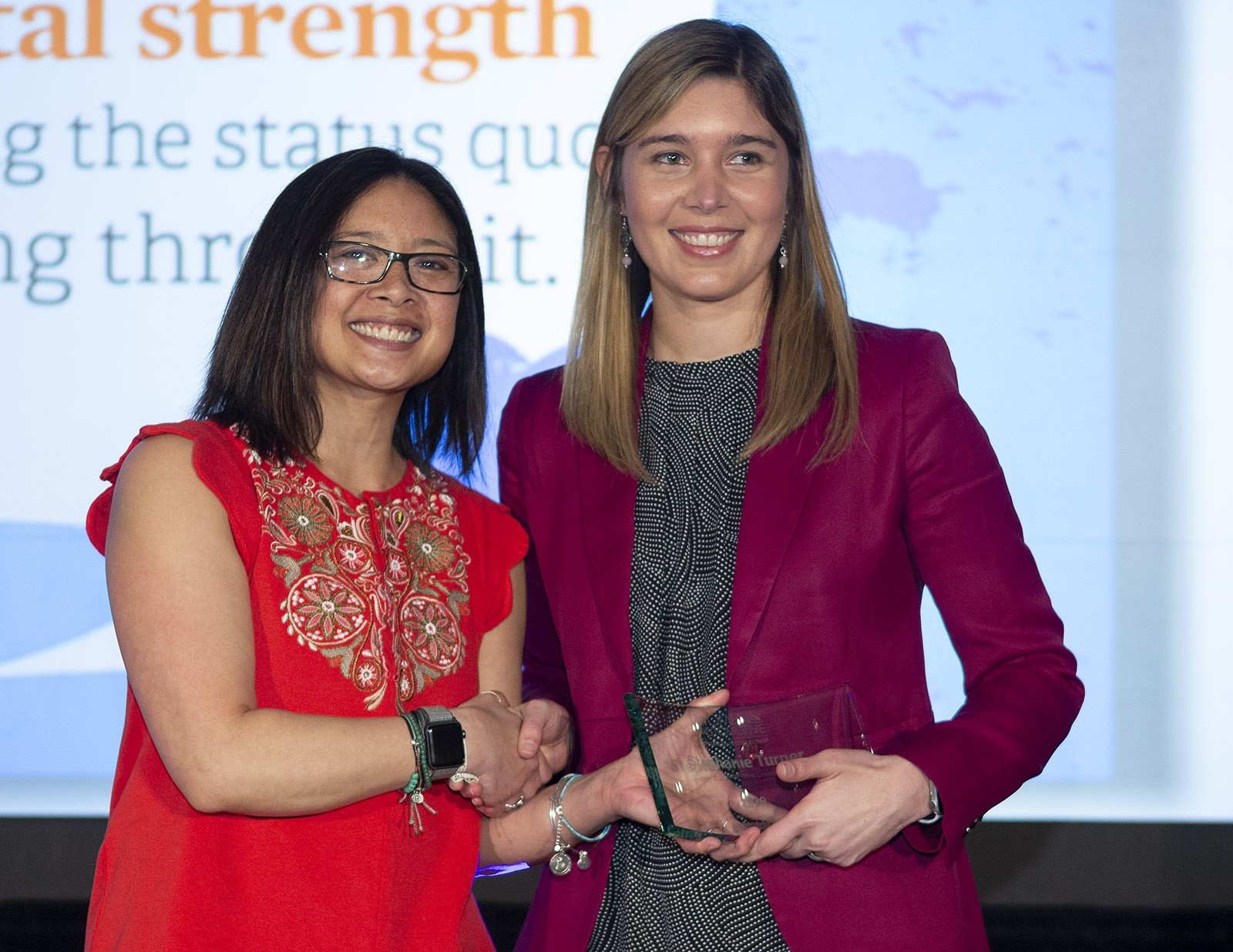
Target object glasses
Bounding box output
[316,240,474,295]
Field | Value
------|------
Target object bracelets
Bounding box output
[451,739,479,783]
[549,774,611,876]
[397,712,437,836]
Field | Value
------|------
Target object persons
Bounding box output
[80,148,790,952]
[450,19,1087,952]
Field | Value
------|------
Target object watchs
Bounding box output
[418,705,466,781]
[917,780,944,824]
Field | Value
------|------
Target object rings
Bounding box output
[480,691,502,704]
[723,816,734,829]
[705,826,720,833]
[505,795,525,808]
[807,853,822,861]
